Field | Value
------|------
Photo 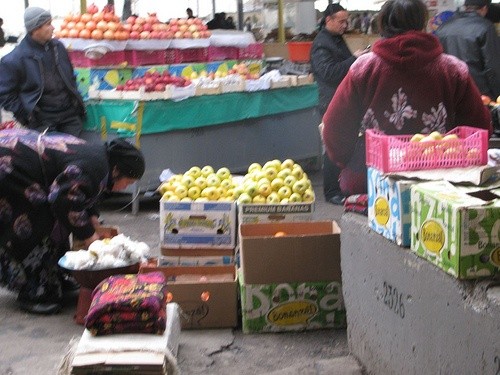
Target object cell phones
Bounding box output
[363,44,371,51]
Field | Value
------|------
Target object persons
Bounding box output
[187,8,192,18]
[0,7,87,137]
[433,0,500,103]
[319,0,493,199]
[207,12,235,29]
[310,3,370,206]
[0,129,145,314]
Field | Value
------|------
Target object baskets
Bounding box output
[209,45,239,61]
[167,48,208,63]
[364,126,487,173]
[68,50,125,68]
[238,42,262,60]
[124,50,168,66]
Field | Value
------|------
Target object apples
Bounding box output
[116,70,192,91]
[480,94,500,107]
[159,159,315,204]
[228,63,260,79]
[409,131,479,160]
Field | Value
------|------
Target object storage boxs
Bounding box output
[409,179,500,281]
[68,50,128,68]
[135,265,240,330]
[158,196,237,250]
[236,193,316,245]
[207,46,240,62]
[156,247,239,266]
[269,73,298,91]
[286,41,313,64]
[238,43,264,60]
[124,47,172,66]
[366,166,433,251]
[166,47,209,64]
[290,73,315,87]
[240,219,341,284]
[195,81,223,97]
[237,268,347,335]
[69,301,182,375]
[72,226,119,251]
[365,124,489,172]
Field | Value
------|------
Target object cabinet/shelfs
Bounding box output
[72,60,323,216]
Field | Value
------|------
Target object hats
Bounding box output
[23,7,51,32]
[318,3,344,31]
[107,138,146,181]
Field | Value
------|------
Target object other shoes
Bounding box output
[16,294,63,313]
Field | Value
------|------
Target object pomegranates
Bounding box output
[54,4,210,40]
